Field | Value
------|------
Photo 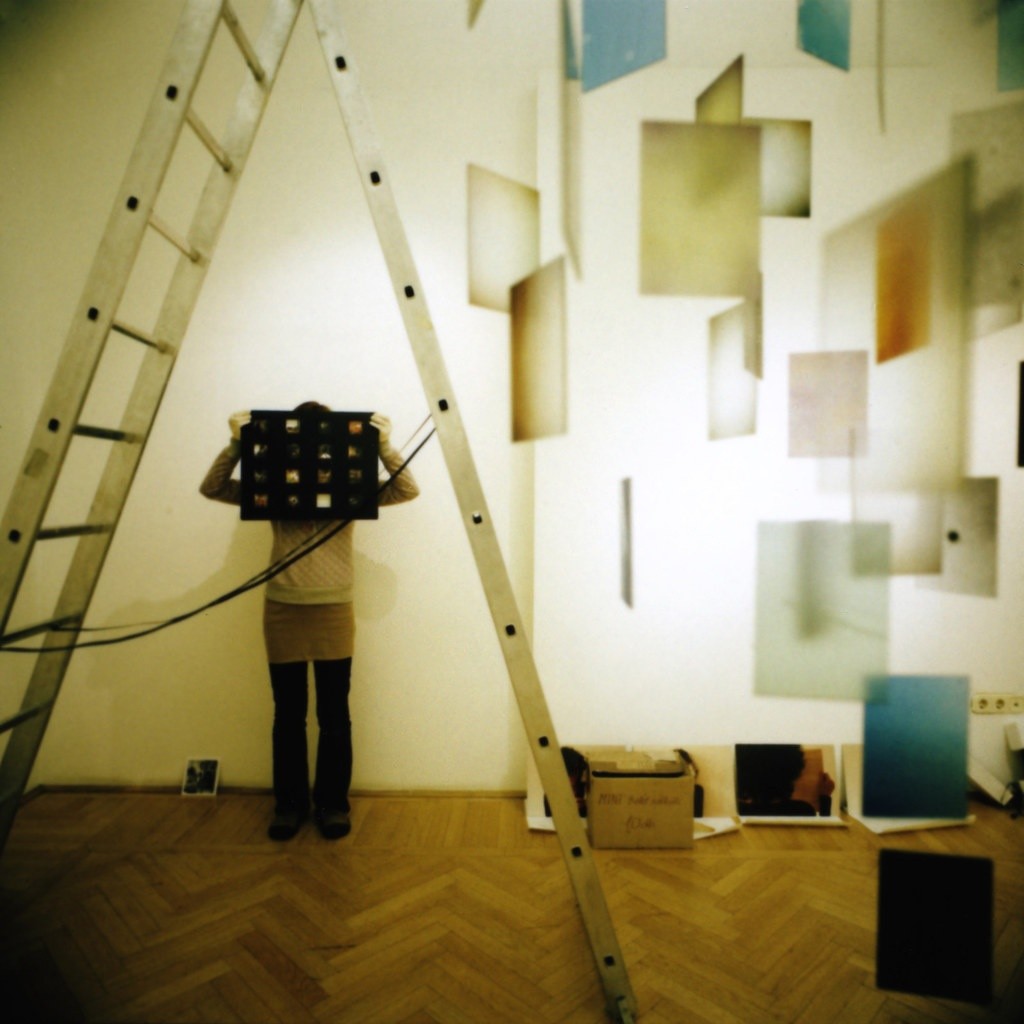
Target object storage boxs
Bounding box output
[587,757,696,849]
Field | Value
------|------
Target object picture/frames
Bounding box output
[181,756,221,797]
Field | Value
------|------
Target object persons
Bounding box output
[198,402,421,842]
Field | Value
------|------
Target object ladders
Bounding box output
[1,0,636,1023]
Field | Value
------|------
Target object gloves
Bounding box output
[367,413,391,452]
[228,410,252,439]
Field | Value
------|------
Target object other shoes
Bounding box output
[266,816,307,840]
[317,815,350,840]
